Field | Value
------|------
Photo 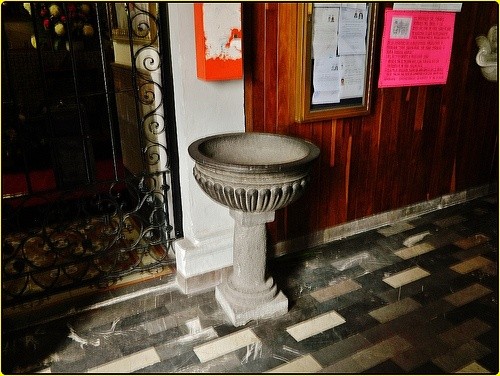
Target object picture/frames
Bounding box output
[106,3,159,47]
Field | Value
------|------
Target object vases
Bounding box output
[30,34,37,49]
[65,41,85,53]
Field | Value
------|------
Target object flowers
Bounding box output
[23,3,94,41]
